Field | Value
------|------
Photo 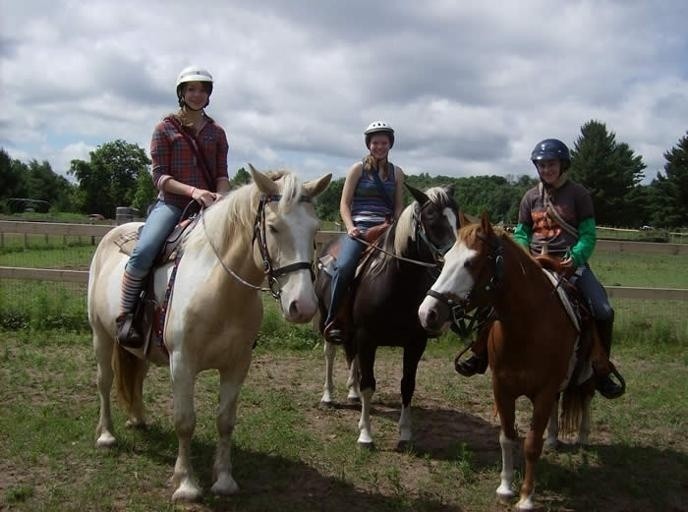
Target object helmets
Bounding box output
[177,66,214,90]
[364,121,394,149]
[531,139,571,165]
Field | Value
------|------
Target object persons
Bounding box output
[456,138,621,394]
[324,120,404,341]
[115,66,230,348]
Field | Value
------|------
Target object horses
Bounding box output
[86,163,333,512]
[418,207,596,512]
[315,182,460,453]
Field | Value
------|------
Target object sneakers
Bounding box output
[456,354,488,375]
[114,316,142,348]
[593,353,611,376]
[328,328,342,339]
